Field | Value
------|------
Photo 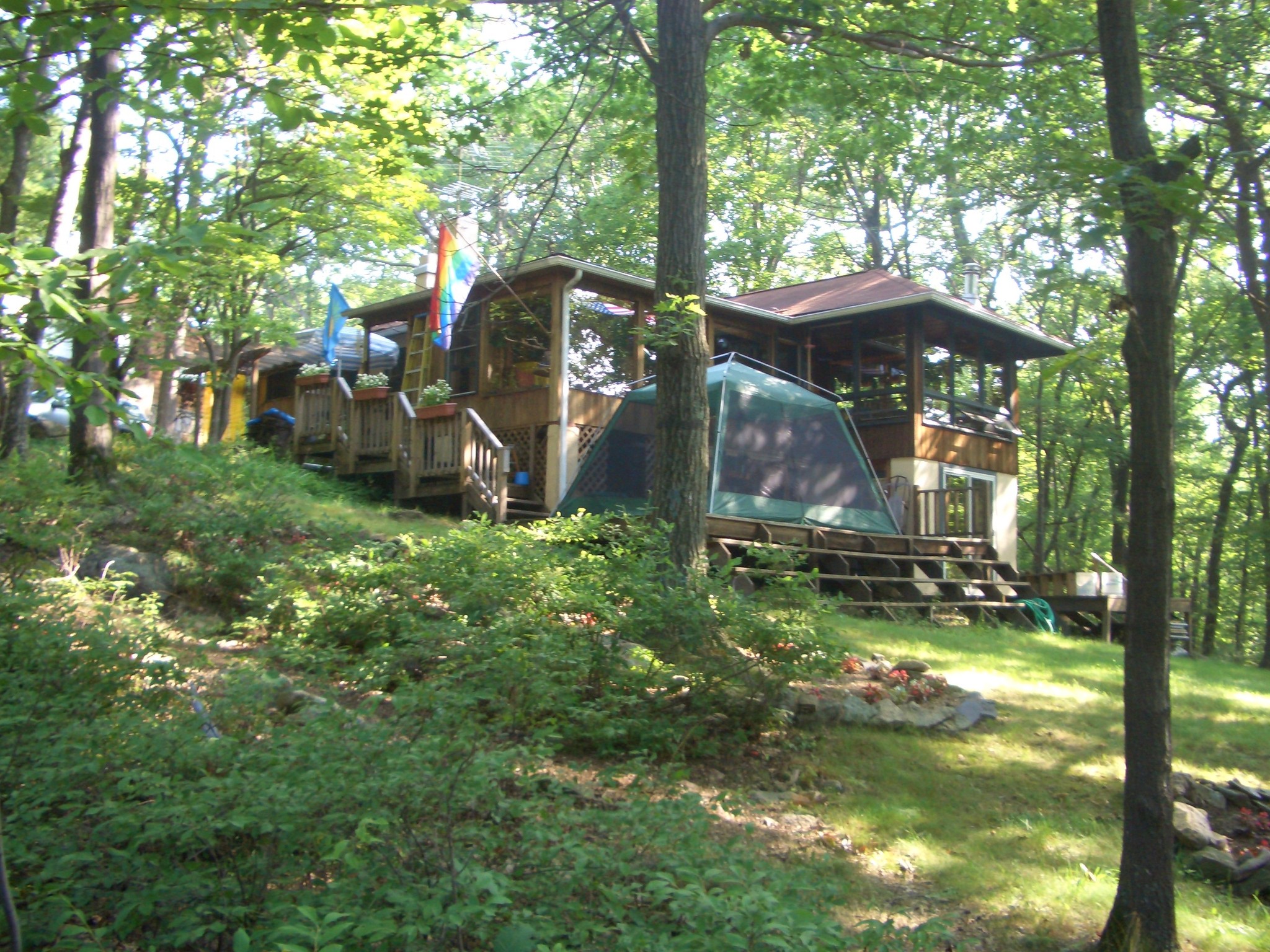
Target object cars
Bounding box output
[26,387,149,442]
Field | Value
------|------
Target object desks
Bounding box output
[1168,594,1192,656]
[1042,594,1127,645]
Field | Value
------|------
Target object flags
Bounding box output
[429,221,480,351]
[322,283,353,367]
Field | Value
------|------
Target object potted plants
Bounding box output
[412,378,458,419]
[351,372,392,400]
[294,362,332,385]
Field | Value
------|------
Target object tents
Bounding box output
[546,354,905,539]
